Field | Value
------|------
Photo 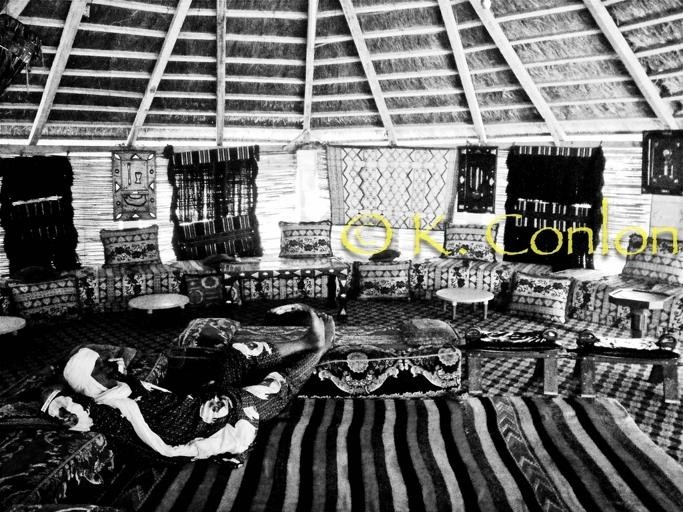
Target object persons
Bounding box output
[660,149,679,178]
[39,306,336,469]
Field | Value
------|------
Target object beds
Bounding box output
[1,348,683,507]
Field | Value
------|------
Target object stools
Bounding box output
[569,329,683,404]
[464,328,565,396]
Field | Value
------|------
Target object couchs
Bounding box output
[162,325,475,399]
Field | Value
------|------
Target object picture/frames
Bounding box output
[641,129,683,196]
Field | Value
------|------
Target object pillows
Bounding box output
[100,224,162,268]
[358,261,408,299]
[440,223,499,262]
[619,234,683,285]
[280,220,333,258]
[5,277,83,325]
[400,318,460,346]
[505,271,574,326]
[178,317,240,350]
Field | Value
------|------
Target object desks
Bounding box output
[129,294,190,315]
[0,316,27,337]
[610,287,675,338]
[437,289,494,321]
